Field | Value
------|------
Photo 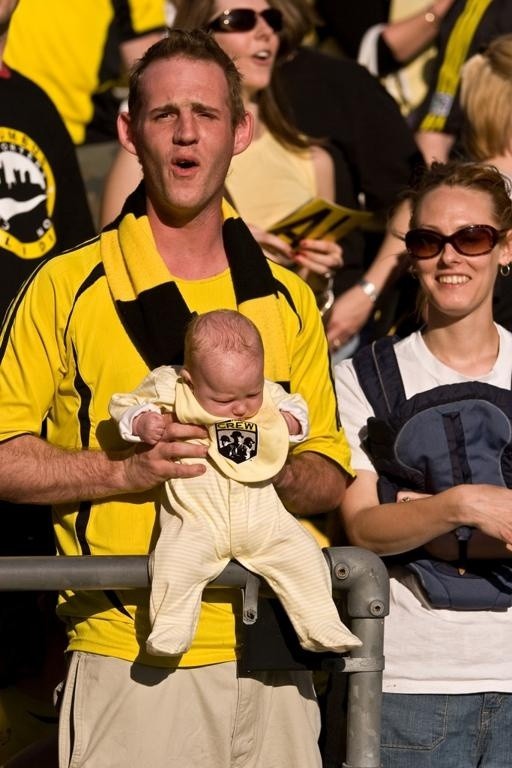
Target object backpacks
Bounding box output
[352,335,512,610]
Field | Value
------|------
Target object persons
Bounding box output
[0,2,512,360]
[329,162,511,767]
[0,34,358,768]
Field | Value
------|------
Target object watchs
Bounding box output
[455,525,472,560]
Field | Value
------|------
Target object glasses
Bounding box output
[405,225,512,258]
[208,6,284,31]
